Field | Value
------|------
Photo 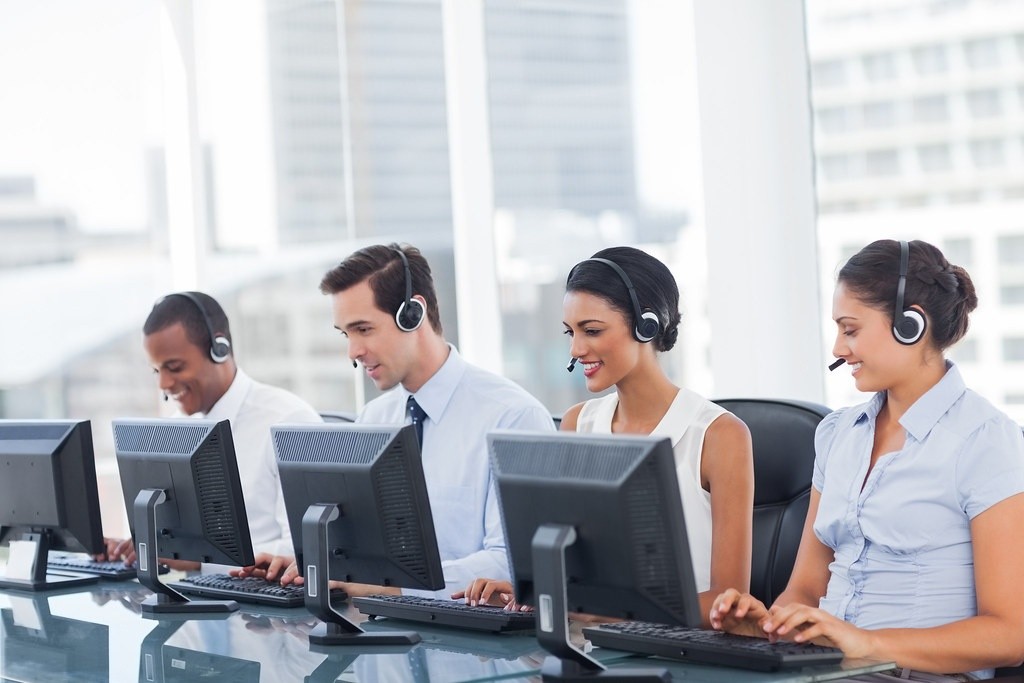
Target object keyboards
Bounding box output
[46,555,170,580]
[581,621,844,671]
[359,618,545,662]
[351,595,537,633]
[165,573,348,607]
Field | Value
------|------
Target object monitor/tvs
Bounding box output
[270,423,446,646]
[485,429,703,683]
[138,613,261,683]
[111,419,257,613]
[0,418,106,593]
[0,587,109,683]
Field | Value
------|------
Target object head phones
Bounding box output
[152,293,231,401]
[352,246,427,368]
[829,240,927,371]
[566,258,660,372]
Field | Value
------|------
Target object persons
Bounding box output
[711,239,1024,683]
[451,247,755,631]
[92,290,327,575]
[229,244,557,604]
[90,588,601,683]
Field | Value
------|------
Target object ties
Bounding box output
[407,395,427,450]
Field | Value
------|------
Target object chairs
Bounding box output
[713,396,830,601]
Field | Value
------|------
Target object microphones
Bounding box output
[164,390,168,400]
[829,357,845,370]
[352,360,357,368]
[566,357,579,372]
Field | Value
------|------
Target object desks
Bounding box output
[0,540,895,683]
